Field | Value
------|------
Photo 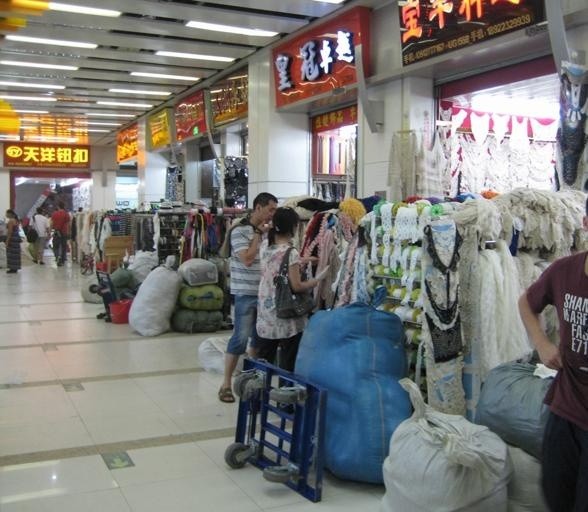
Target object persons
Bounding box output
[252,205,319,415]
[1,199,84,274]
[217,193,278,403]
[518,196,588,510]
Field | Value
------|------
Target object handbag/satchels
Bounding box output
[273,275,317,318]
[27,229,39,244]
[219,215,264,259]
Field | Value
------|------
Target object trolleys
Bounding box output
[222,354,328,502]
[87,269,116,321]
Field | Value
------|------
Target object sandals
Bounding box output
[219,386,235,402]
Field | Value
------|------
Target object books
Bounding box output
[308,264,330,293]
